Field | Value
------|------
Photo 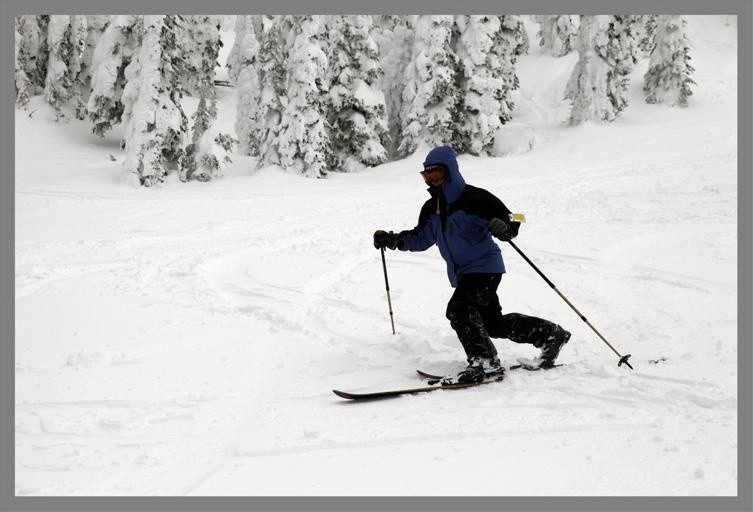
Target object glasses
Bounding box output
[420,167,447,183]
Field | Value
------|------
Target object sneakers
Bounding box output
[451,356,503,384]
[536,325,571,364]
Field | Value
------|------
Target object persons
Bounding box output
[372,145,572,388]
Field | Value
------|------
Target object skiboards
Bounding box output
[333,359,666,399]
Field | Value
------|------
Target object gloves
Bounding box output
[486,218,508,236]
[374,230,402,249]
